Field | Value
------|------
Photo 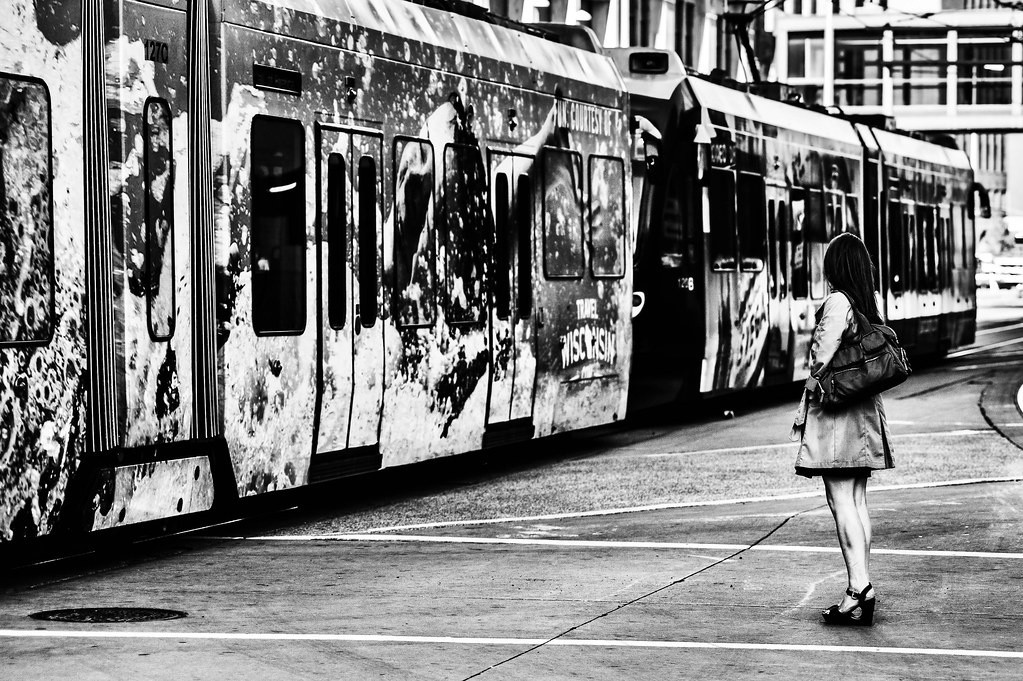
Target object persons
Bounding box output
[787,232,897,627]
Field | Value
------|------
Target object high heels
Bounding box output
[822,582,875,627]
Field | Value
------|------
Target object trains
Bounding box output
[1,2,993,582]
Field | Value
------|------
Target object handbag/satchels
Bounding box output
[812,289,911,417]
